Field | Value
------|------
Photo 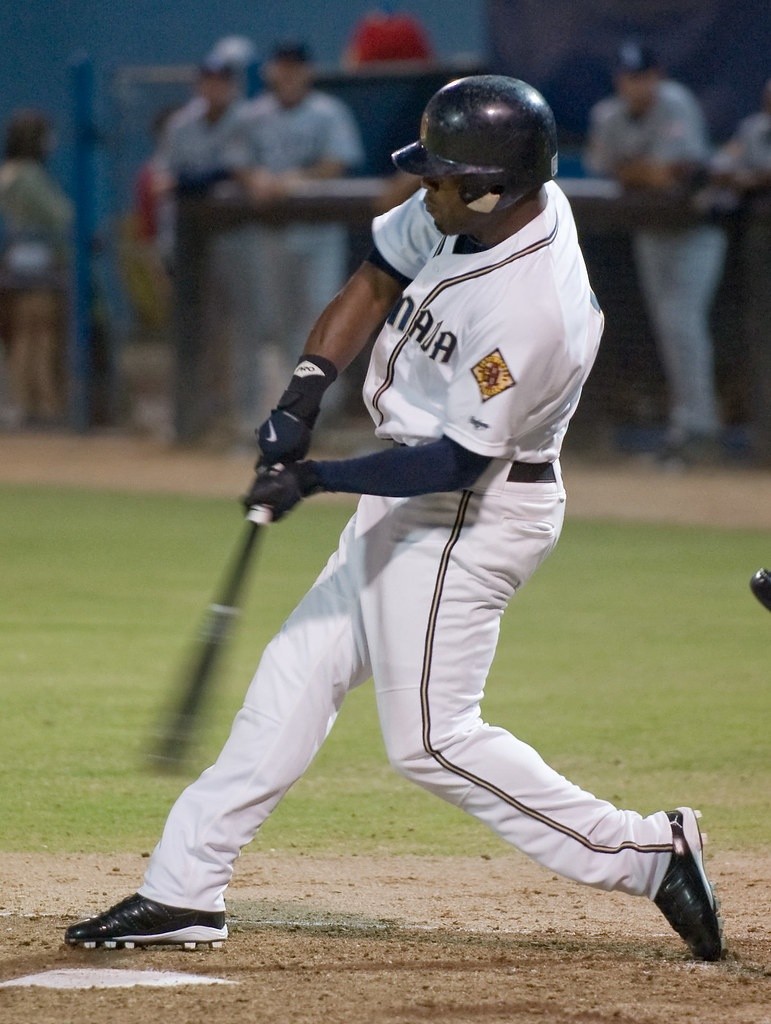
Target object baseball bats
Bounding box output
[141,462,295,787]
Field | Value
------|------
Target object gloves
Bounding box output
[259,396,318,466]
[244,462,320,522]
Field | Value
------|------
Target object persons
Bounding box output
[582,38,771,456]
[140,34,365,453]
[0,108,76,428]
[341,0,439,74]
[749,567,771,611]
[65,75,724,962]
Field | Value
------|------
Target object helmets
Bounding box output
[391,71,559,213]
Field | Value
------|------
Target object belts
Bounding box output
[508,460,561,484]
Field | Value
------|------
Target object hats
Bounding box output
[268,36,314,66]
[613,43,661,76]
[199,49,244,80]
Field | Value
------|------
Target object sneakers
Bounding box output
[651,806,723,960]
[65,885,228,954]
